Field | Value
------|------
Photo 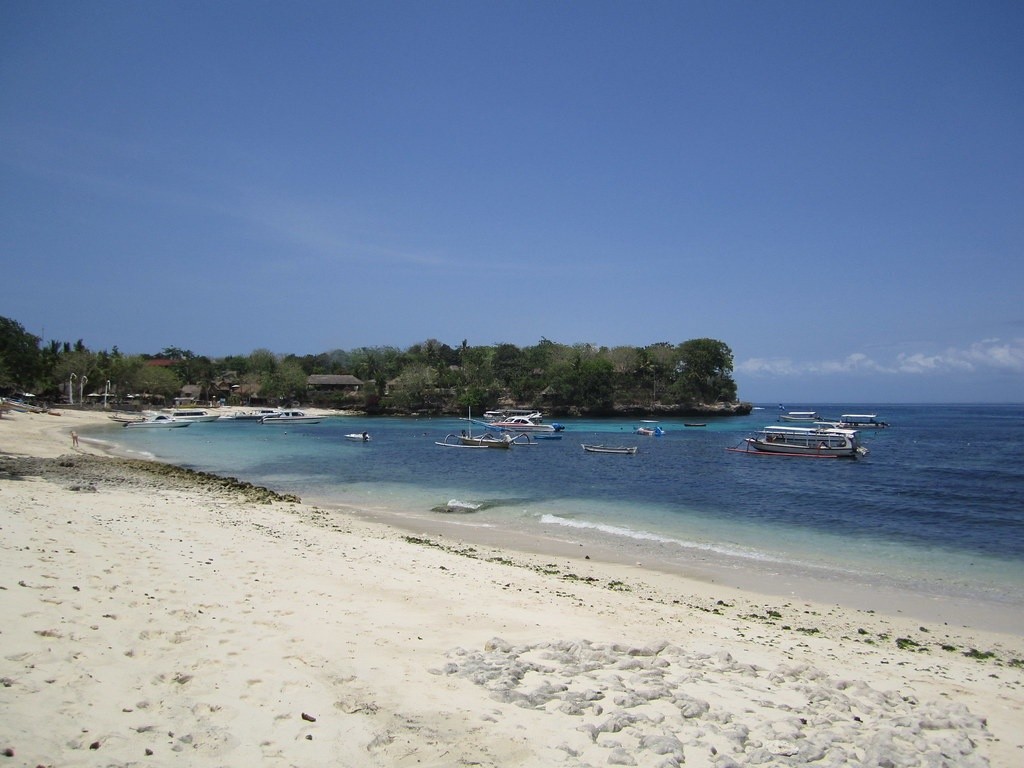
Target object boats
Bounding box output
[636,419,666,436]
[124,414,196,428]
[580,443,638,454]
[812,414,885,429]
[533,435,563,440]
[744,425,860,458]
[684,423,706,427]
[779,411,818,423]
[233,409,283,419]
[172,411,221,422]
[456,434,510,448]
[482,410,544,423]
[490,415,556,432]
[260,411,330,424]
[345,434,372,442]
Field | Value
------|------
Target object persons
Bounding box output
[363,431,368,440]
[70,430,79,447]
